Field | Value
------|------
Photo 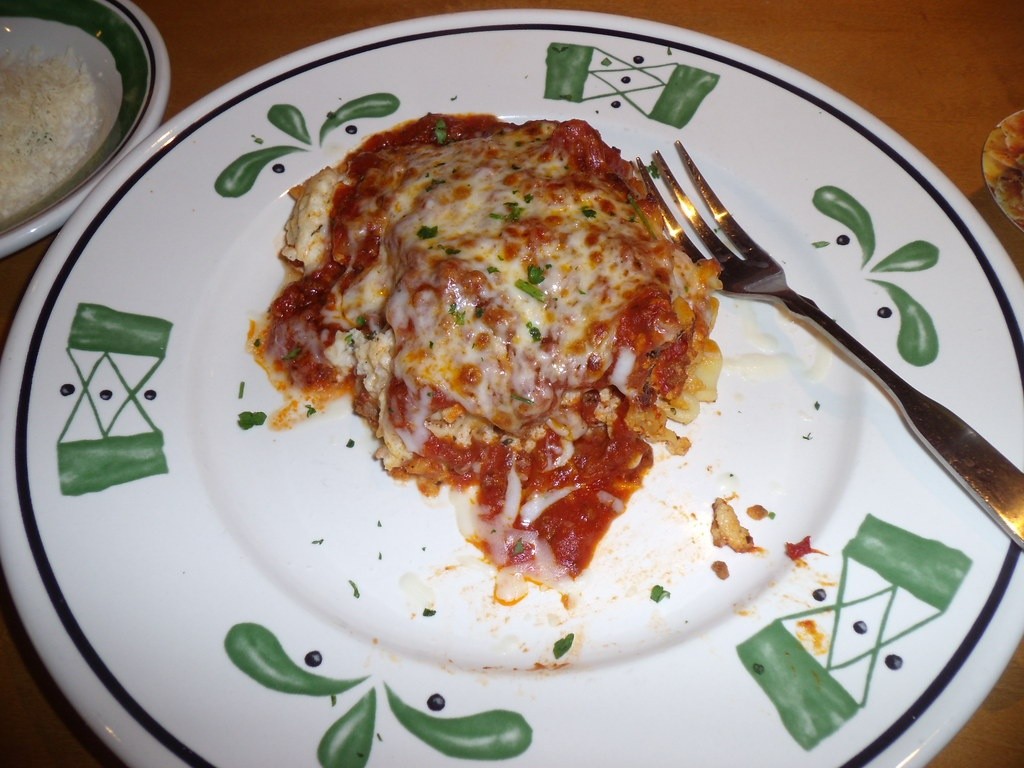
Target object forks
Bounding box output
[628,142,1022,547]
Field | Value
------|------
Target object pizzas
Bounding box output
[257,109,724,588]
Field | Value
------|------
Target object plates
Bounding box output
[0,5,1022,768]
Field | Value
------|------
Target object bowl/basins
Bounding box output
[0,1,171,260]
